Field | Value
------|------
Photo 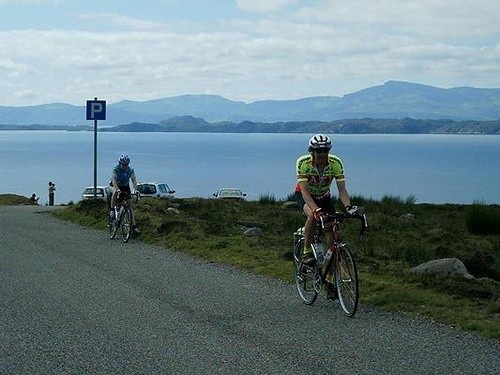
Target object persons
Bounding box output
[48,182,55,207]
[30,194,39,204]
[109,154,143,234]
[294,134,360,283]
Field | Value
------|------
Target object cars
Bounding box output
[213,188,247,202]
[82,186,112,204]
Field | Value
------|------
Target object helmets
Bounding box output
[119,154,130,164]
[308,134,332,152]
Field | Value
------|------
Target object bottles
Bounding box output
[119,207,123,217]
[314,240,324,262]
[322,246,333,263]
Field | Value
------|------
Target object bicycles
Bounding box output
[292,207,369,318]
[109,190,140,242]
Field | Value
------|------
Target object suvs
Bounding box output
[134,182,176,199]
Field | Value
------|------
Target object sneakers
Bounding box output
[324,282,343,300]
[302,251,316,265]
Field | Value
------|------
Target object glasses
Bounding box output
[120,164,128,167]
[312,148,330,154]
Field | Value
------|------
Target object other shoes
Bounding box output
[132,227,139,233]
[109,210,114,217]
